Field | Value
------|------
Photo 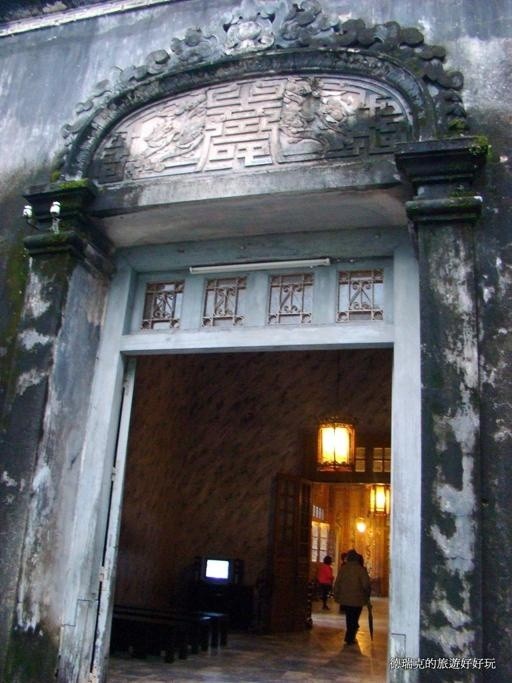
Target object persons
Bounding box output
[334,549,372,645]
[316,555,332,611]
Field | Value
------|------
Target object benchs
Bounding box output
[110,605,229,663]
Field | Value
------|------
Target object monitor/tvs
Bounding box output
[201,555,248,584]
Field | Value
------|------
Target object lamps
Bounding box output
[21,199,62,234]
[367,484,391,517]
[314,351,358,474]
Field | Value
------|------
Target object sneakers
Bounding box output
[344,637,358,645]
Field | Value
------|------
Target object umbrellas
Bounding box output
[366,601,374,642]
